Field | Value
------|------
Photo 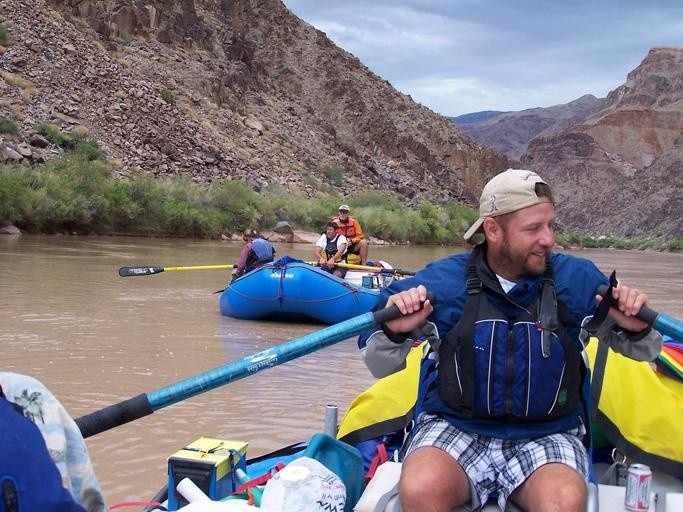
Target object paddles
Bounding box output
[119,265,238,276]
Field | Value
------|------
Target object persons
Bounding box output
[331,204,369,267]
[359,169,662,511]
[232,229,276,280]
[314,221,348,278]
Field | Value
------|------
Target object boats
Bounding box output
[147,337,683,505]
[218,260,397,325]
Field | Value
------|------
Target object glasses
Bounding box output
[340,210,349,213]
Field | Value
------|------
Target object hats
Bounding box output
[243,229,258,241]
[462,167,556,241]
[339,204,351,212]
